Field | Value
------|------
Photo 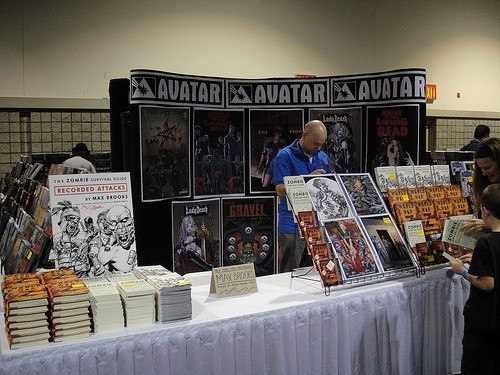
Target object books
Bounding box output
[0,158,193,351]
[374,160,478,267]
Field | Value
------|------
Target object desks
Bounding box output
[5,263,472,375]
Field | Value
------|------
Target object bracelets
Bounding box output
[463,269,468,278]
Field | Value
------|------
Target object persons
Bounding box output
[273,120,332,274]
[449,125,500,375]
[63,143,96,173]
